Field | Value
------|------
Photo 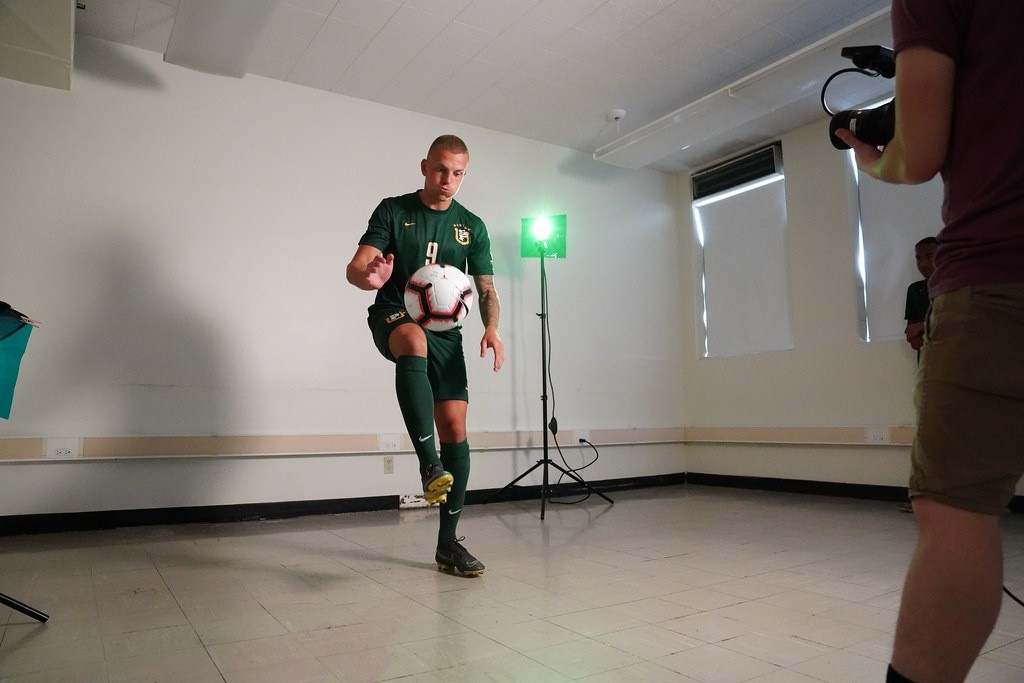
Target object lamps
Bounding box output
[491,215,613,522]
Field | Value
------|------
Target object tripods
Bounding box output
[487,258,615,524]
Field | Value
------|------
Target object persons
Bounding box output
[903,236,940,365]
[346,134,506,577]
[834,0,1024,682]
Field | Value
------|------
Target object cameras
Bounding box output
[821,45,896,148]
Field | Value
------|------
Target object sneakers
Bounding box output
[435,537,484,576]
[421,463,453,507]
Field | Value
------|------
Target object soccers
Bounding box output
[403,263,474,332]
